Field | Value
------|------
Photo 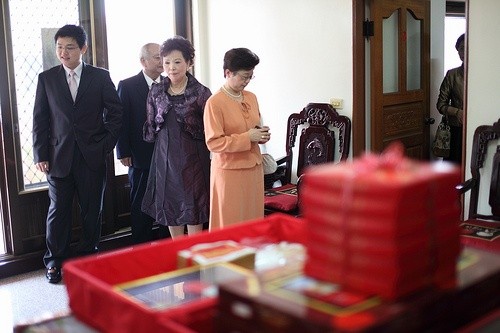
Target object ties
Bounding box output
[68,71,77,102]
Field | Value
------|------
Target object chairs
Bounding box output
[260,103,352,219]
[456,118,500,254]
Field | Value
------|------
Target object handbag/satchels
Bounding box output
[432,111,452,158]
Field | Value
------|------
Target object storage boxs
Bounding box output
[64,156,500,333]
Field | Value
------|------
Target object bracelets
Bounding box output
[456,109,459,117]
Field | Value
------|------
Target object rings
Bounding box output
[262,136,264,138]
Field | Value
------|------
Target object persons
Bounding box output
[203,48,271,232]
[116,43,166,243]
[436,33,463,163]
[32,25,124,283]
[141,36,212,238]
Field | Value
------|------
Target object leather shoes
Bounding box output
[45,267,62,283]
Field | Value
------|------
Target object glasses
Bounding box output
[235,72,255,80]
[56,44,78,49]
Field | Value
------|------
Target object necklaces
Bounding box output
[170,76,188,94]
[222,85,241,98]
